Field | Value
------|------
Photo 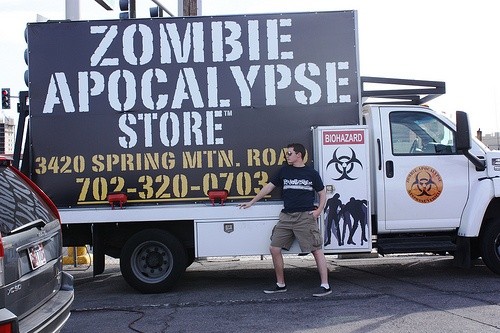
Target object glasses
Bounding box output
[285,151,297,156]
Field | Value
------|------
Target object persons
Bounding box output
[236,143,333,297]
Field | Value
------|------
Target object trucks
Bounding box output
[25,8,500,294]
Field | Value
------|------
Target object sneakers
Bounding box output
[264,283,287,293]
[312,287,332,296]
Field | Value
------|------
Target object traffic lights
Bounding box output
[1,88,10,109]
[118,0,137,19]
[149,6,160,18]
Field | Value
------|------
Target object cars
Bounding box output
[0,155,76,333]
